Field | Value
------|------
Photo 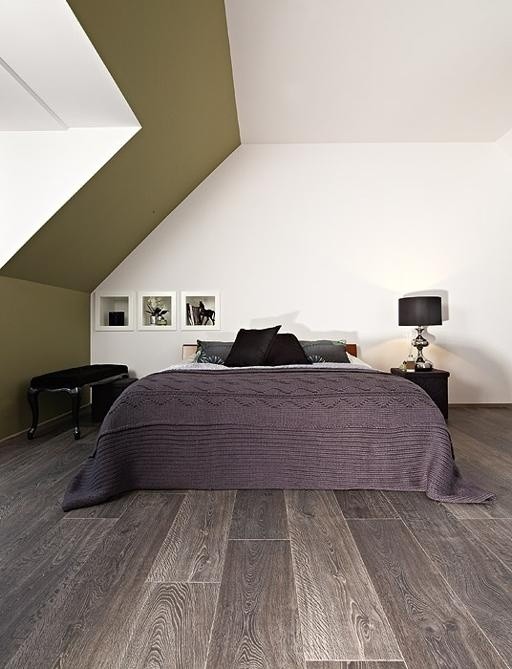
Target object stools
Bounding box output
[89,376,138,423]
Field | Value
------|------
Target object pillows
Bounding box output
[199,338,232,365]
[272,334,311,363]
[226,328,282,366]
[299,338,351,364]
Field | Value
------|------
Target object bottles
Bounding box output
[399,354,416,373]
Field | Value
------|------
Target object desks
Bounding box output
[27,364,130,440]
[390,367,450,420]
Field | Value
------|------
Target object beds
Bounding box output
[61,344,495,509]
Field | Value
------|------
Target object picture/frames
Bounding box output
[181,291,220,331]
[138,290,176,330]
[94,295,134,331]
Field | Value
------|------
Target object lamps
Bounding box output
[398,297,443,369]
